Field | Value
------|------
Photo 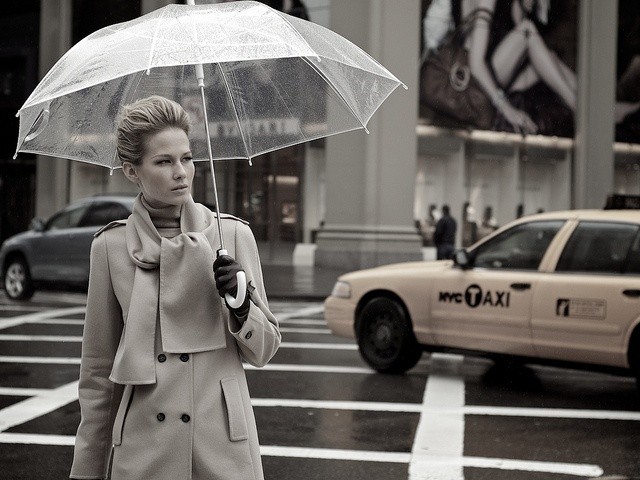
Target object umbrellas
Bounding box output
[13,0,407,308]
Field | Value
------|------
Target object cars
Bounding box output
[325,207,640,374]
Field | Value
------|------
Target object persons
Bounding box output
[464,201,474,221]
[68,95,282,479]
[427,203,439,224]
[481,205,498,229]
[432,204,456,260]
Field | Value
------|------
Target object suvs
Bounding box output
[0,196,135,300]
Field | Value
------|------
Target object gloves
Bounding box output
[214,255,252,316]
